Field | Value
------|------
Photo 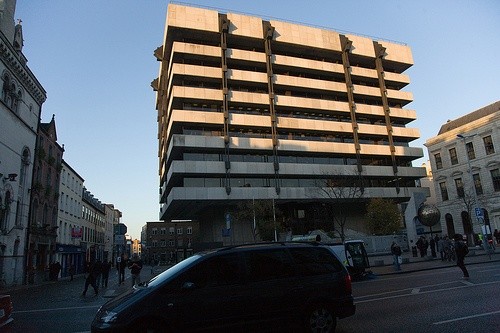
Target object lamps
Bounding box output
[5,173,18,181]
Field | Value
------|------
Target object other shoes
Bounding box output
[460,277,468,280]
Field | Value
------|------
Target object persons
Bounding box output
[463,233,483,247]
[454,234,469,277]
[83,258,111,295]
[416,234,454,261]
[116,254,143,287]
[50,261,74,281]
[393,243,402,270]
[494,229,500,243]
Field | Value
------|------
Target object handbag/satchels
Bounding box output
[398,256,403,265]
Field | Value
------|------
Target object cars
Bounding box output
[0,293,15,329]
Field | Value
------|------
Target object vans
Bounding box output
[91,239,357,333]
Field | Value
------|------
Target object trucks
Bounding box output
[320,240,371,282]
